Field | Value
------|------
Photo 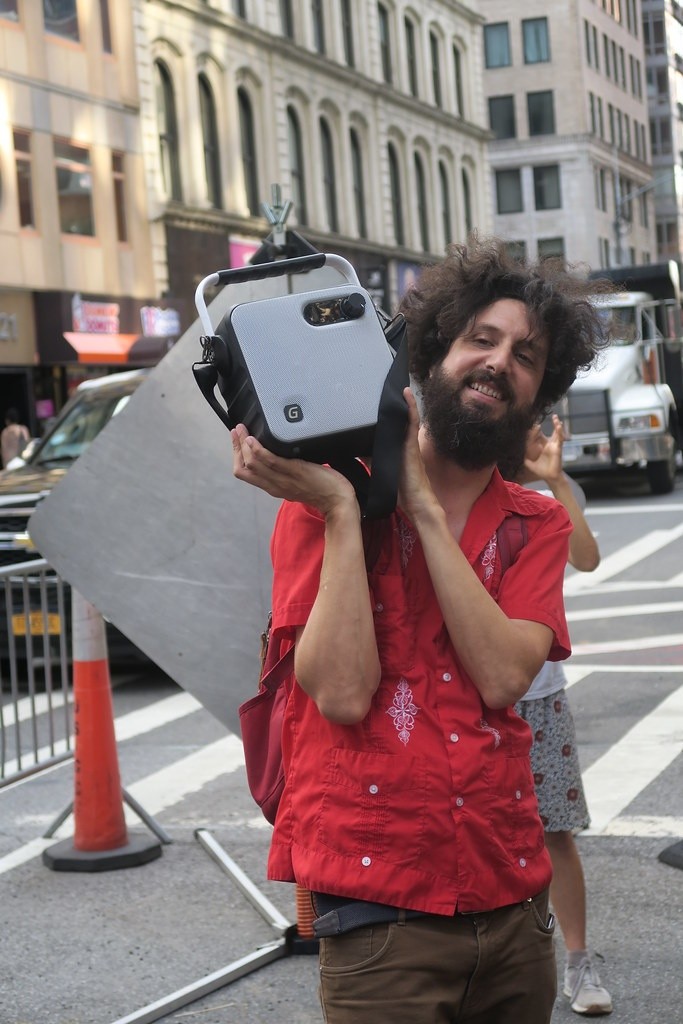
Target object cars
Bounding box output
[0,366,179,689]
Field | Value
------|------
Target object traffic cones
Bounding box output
[42,585,165,875]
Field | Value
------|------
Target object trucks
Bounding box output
[518,259,681,498]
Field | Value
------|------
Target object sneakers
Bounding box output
[563,952,613,1014]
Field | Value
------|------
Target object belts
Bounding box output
[312,893,494,938]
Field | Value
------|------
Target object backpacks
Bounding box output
[239,461,528,828]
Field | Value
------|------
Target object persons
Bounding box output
[231,245,587,1024]
[0,407,31,470]
[513,410,615,1018]
